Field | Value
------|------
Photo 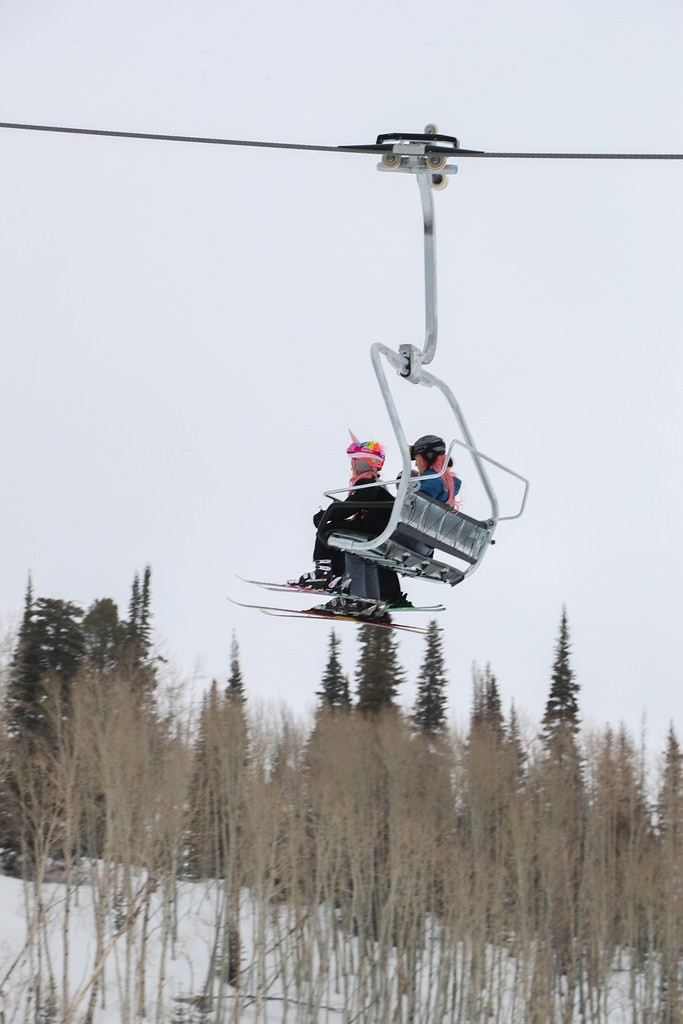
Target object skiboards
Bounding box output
[229,579,447,638]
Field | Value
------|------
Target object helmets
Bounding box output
[347,440,385,471]
[410,434,446,464]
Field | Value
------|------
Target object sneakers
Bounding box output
[298,558,334,585]
[325,594,392,622]
[380,592,412,608]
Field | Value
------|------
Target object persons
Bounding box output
[298,442,398,587]
[323,435,461,615]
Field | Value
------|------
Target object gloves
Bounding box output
[314,510,325,528]
